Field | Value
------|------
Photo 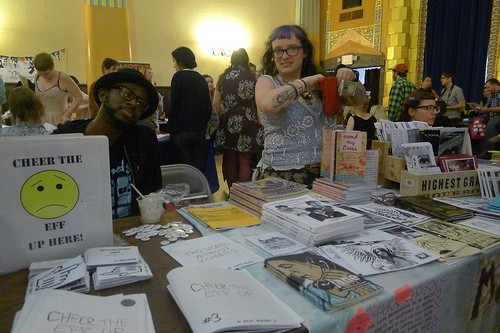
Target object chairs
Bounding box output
[162,164,214,209]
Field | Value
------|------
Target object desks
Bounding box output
[155,134,220,195]
[0,162,500,333]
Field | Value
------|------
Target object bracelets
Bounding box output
[288,78,308,100]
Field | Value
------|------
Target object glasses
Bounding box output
[270,45,302,58]
[112,85,152,112]
[413,104,440,114]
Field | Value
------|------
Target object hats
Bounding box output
[93,67,160,121]
[15,79,35,90]
[393,63,408,78]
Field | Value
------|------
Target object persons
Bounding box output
[339,92,379,149]
[0,86,51,136]
[32,52,84,128]
[388,64,417,121]
[303,200,347,222]
[202,73,220,149]
[210,48,265,196]
[251,25,369,191]
[408,74,500,162]
[166,46,212,174]
[69,55,163,136]
[52,67,163,219]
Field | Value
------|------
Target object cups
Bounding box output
[137,195,163,224]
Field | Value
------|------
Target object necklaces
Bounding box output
[282,77,314,105]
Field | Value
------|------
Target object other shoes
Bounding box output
[477,152,490,159]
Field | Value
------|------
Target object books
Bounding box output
[11,123,500,333]
